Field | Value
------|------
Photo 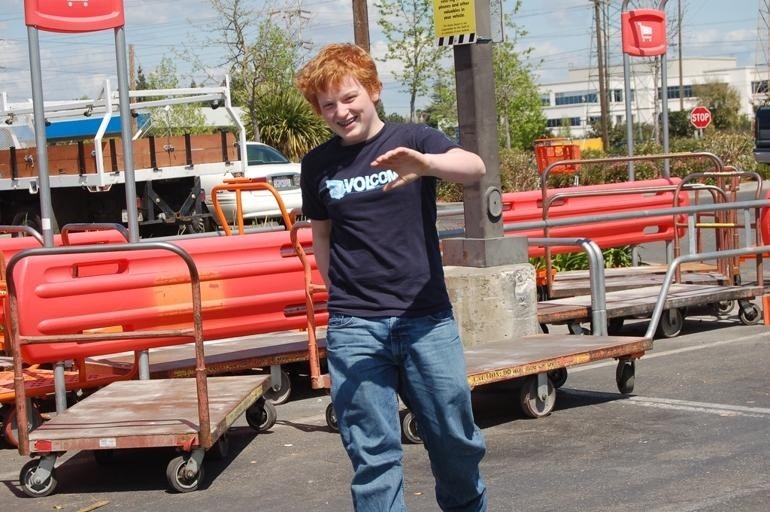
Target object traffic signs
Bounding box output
[690,107,712,129]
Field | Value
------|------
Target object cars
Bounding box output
[198,140,309,232]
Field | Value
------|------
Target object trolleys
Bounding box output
[4,237,278,499]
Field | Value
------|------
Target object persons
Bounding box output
[294,43,490,512]
